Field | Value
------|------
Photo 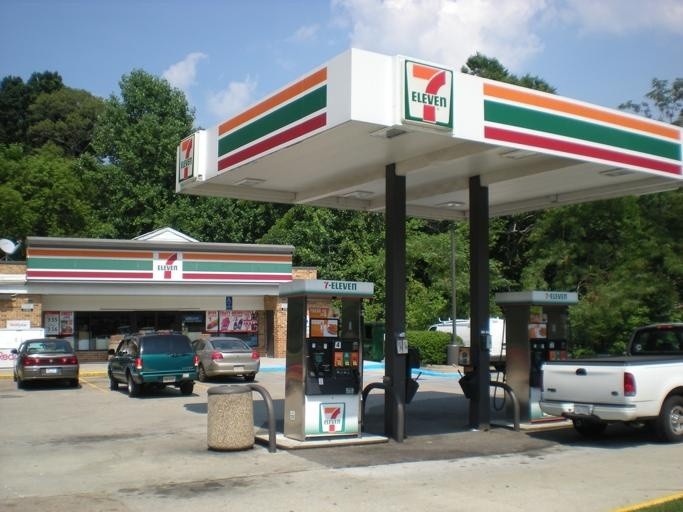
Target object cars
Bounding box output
[192,337,261,382]
[10,338,79,387]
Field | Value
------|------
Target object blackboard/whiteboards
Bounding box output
[1,328,44,349]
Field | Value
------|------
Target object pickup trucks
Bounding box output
[541,322,683,442]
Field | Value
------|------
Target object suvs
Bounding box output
[106,333,197,396]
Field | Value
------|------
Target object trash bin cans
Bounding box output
[447,345,460,366]
[207,385,254,451]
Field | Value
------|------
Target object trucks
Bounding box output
[428,315,508,375]
